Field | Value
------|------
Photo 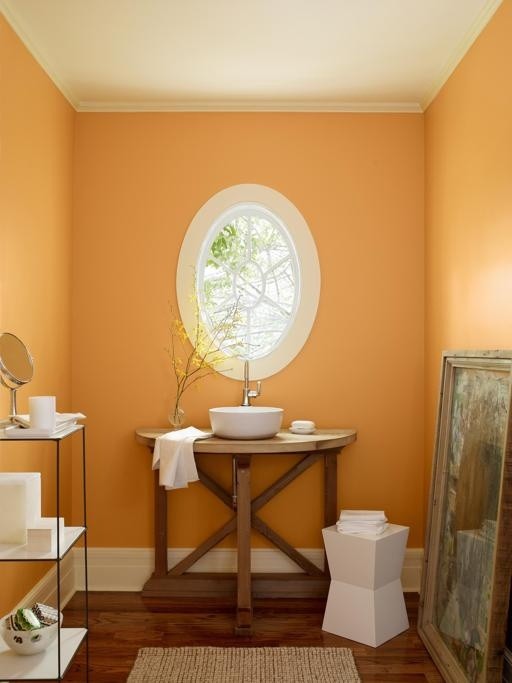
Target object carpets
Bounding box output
[125,644,362,682]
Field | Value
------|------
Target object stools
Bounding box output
[321,522,412,649]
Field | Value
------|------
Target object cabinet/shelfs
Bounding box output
[2,423,93,683]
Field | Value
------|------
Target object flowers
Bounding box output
[166,261,258,425]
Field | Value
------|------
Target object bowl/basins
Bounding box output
[1,609,64,655]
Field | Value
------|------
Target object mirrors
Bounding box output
[1,330,37,429]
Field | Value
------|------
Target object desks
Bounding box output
[132,427,360,641]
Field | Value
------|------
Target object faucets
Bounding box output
[241,359,261,406]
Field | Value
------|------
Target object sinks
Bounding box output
[208,406,284,440]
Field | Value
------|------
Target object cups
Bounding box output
[290,421,315,431]
[28,396,56,437]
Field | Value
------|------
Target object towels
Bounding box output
[8,411,87,435]
[335,506,391,537]
[148,422,215,494]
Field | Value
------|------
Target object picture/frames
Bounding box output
[414,344,512,682]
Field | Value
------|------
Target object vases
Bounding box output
[168,395,186,430]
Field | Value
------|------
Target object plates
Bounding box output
[289,427,316,434]
